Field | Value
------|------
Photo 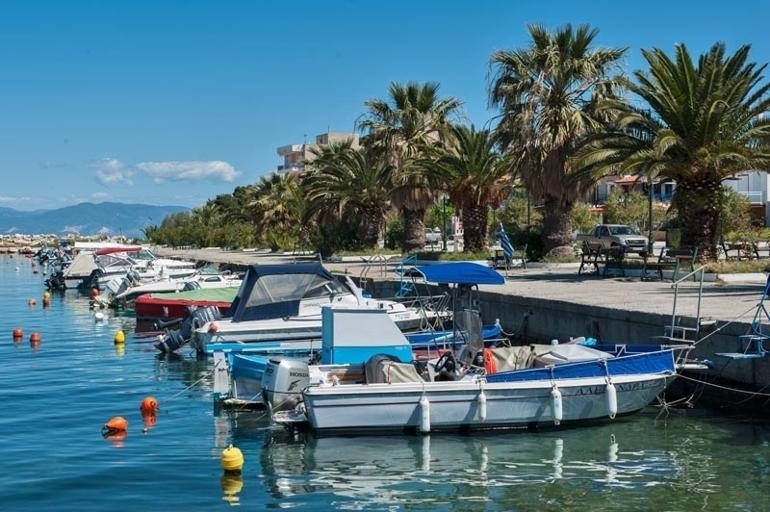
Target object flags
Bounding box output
[499,222,514,269]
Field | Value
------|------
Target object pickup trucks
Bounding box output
[577,224,649,257]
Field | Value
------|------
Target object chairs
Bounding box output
[577,240,698,283]
